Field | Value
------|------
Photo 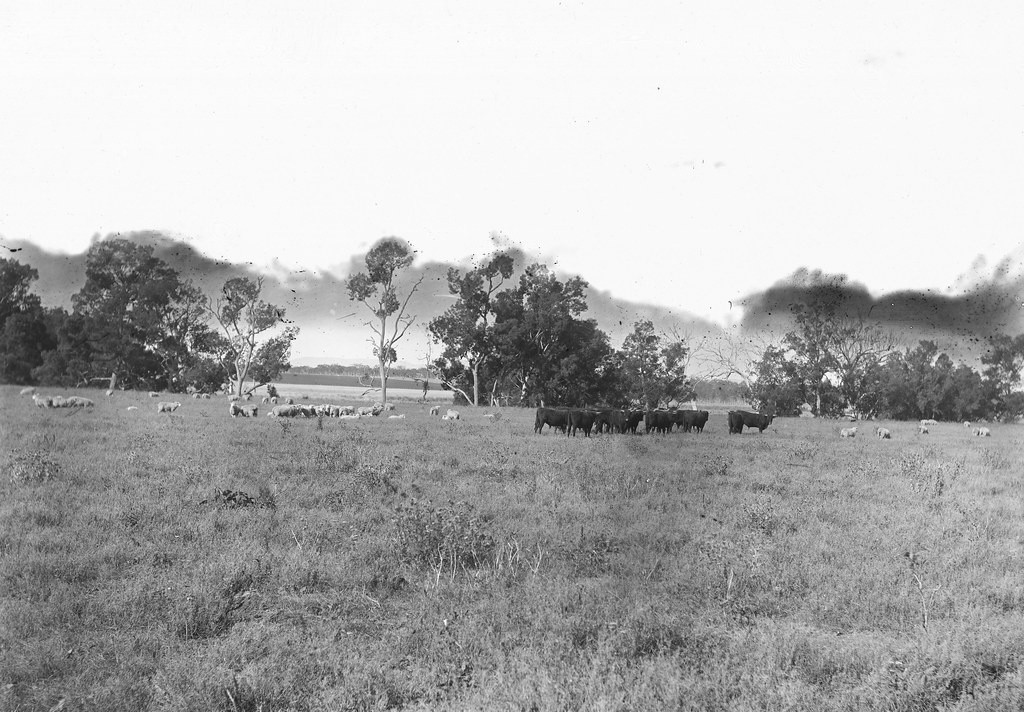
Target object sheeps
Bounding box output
[842,419,989,442]
[20,386,459,422]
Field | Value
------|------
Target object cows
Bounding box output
[536,407,775,436]
